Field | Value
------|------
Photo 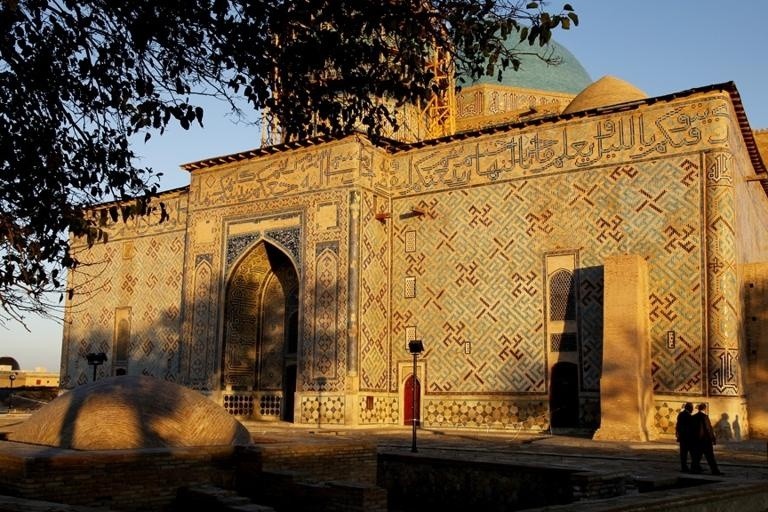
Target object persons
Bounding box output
[687,403,724,478]
[673,400,693,472]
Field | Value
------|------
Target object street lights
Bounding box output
[86,352,108,381]
[409,339,425,453]
[9,375,16,408]
